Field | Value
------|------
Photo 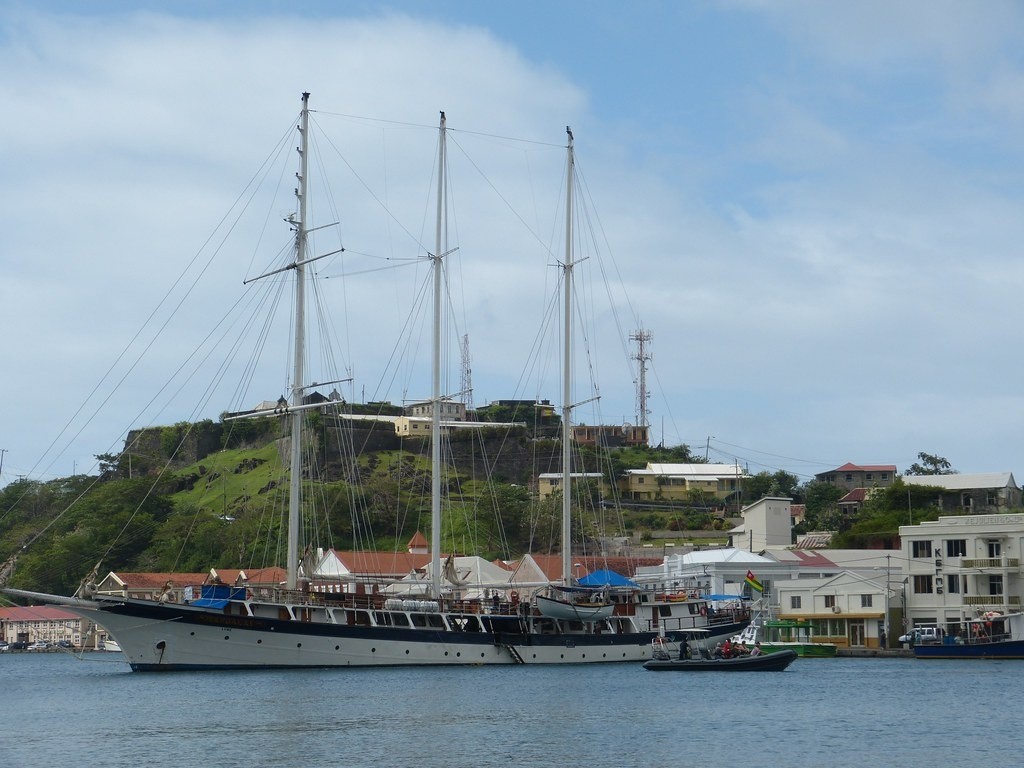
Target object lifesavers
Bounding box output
[693,589,701,599]
[700,606,707,617]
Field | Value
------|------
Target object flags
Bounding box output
[744,569,765,593]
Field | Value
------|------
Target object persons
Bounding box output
[679,638,692,660]
[880,629,886,649]
[909,630,921,649]
[707,605,716,626]
[715,638,769,659]
[954,633,962,644]
[493,592,501,614]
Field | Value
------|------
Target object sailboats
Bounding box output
[1,90,752,675]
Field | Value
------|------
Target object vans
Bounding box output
[898,627,946,644]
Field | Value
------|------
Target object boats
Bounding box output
[721,617,838,658]
[642,625,799,671]
[913,612,1024,660]
